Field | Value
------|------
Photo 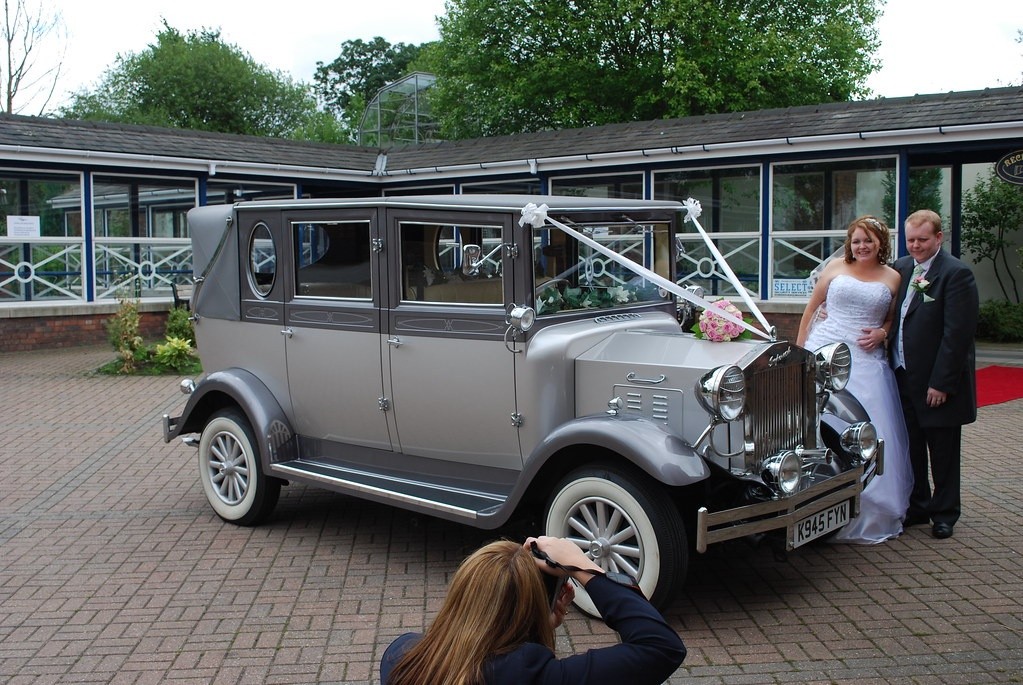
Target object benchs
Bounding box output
[410,274,553,302]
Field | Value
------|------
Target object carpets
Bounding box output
[976,366,1023,409]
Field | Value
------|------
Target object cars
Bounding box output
[162,194,885,625]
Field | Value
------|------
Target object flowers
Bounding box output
[910,274,930,300]
[420,262,502,288]
[689,297,754,342]
[535,281,670,315]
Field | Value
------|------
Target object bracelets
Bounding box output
[879,328,887,339]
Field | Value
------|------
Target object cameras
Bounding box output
[540,573,569,615]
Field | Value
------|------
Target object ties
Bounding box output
[906,265,924,296]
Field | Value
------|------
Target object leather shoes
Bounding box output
[932,521,953,538]
[902,515,930,527]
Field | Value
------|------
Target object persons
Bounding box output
[380,536,686,685]
[795,215,915,545]
[817,209,977,539]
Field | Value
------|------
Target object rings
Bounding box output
[565,612,568,615]
[937,400,941,401]
